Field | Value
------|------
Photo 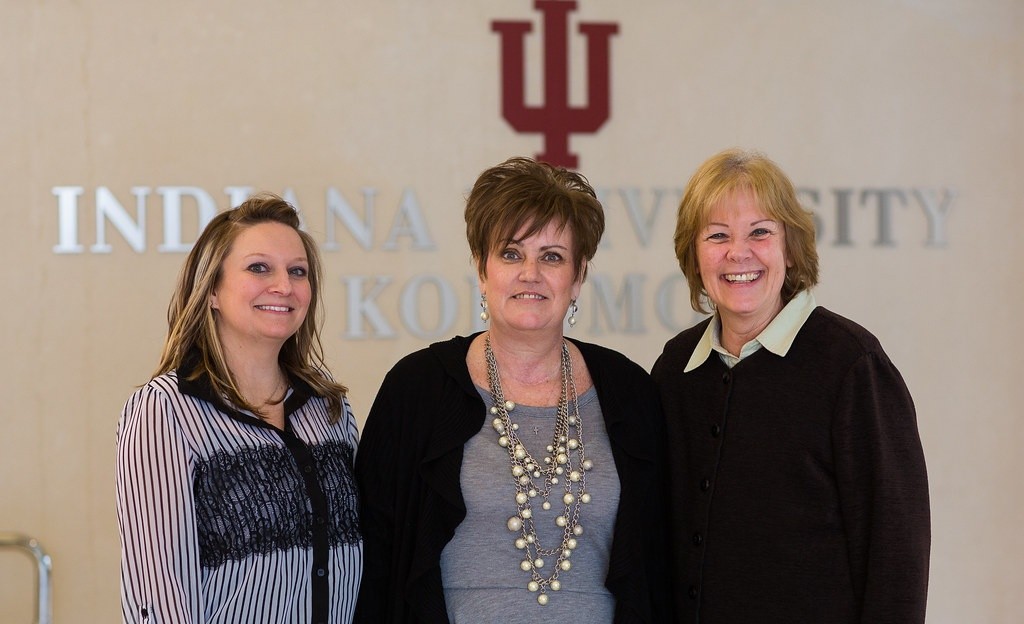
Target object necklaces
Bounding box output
[485,329,593,605]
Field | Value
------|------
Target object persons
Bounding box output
[113,192,362,624]
[349,156,672,624]
[649,149,931,624]
